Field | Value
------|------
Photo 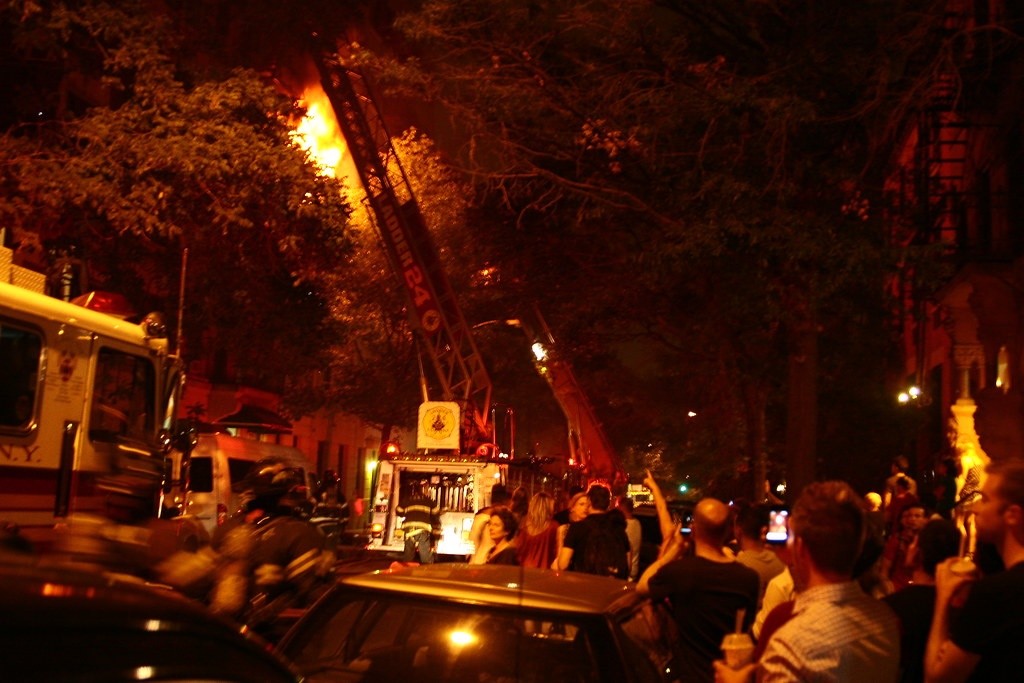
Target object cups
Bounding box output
[949,561,977,608]
[719,633,756,670]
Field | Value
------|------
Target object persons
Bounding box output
[53,456,352,645]
[468,483,642,582]
[395,483,442,565]
[636,455,1024,683]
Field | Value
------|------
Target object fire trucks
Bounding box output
[300,2,655,556]
[0,226,198,519]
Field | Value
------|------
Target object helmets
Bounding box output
[251,456,297,506]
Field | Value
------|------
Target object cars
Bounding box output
[280,564,675,683]
[0,554,301,683]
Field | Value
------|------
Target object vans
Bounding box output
[158,426,314,539]
